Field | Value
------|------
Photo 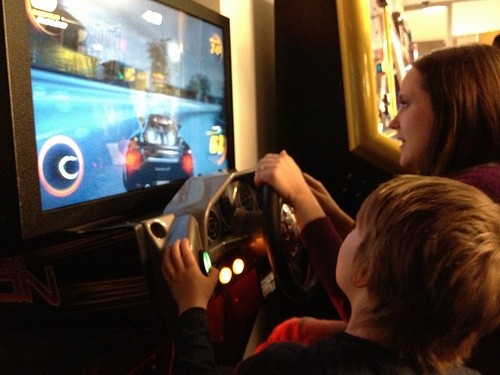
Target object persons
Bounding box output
[159,172,500,374]
[253,43,500,325]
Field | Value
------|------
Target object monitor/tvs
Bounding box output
[0,0,235,238]
